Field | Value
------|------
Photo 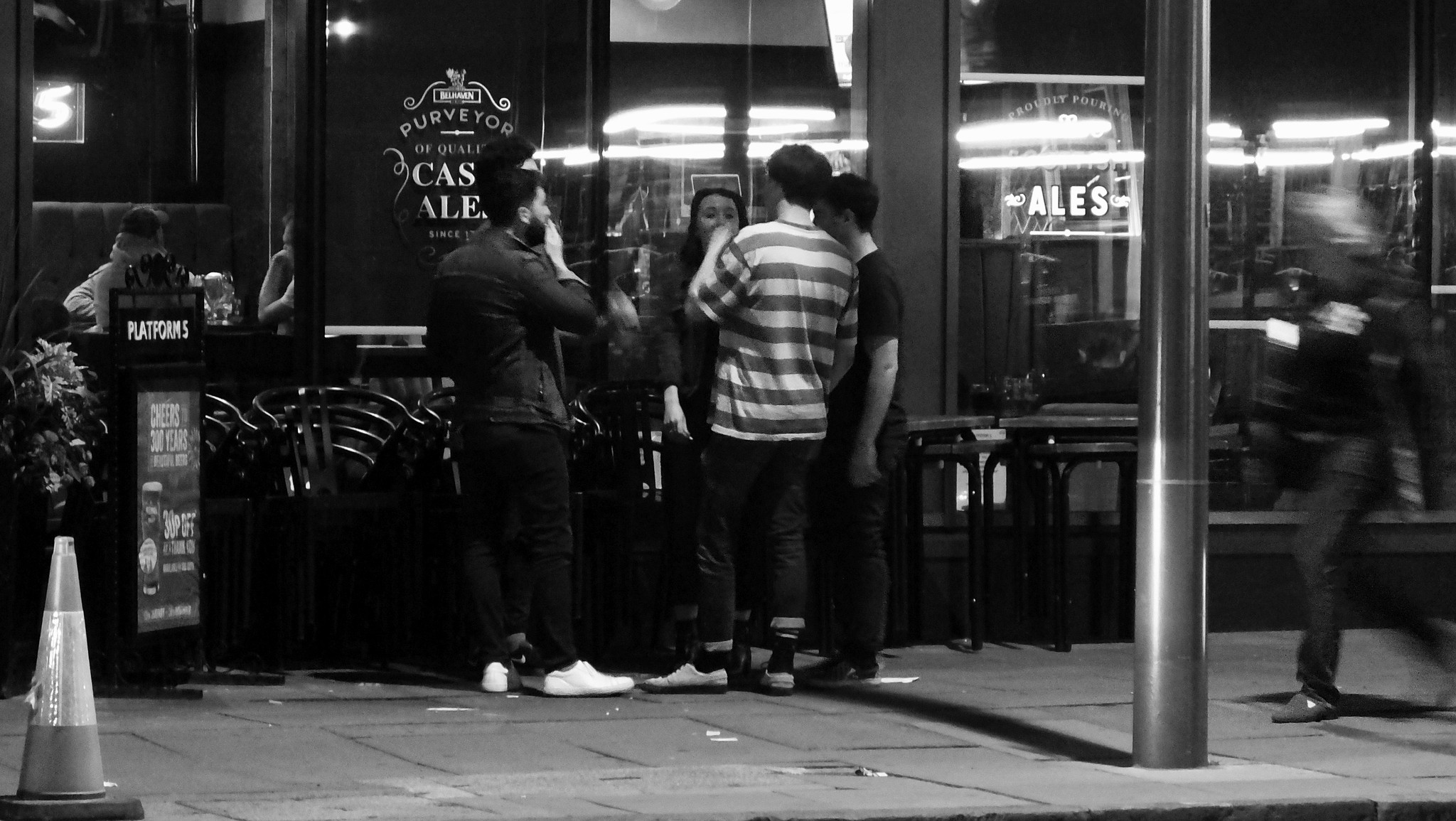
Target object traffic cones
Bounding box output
[0,536,145,821]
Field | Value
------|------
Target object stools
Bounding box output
[895,404,1235,656]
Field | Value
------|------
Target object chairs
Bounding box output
[180,376,691,676]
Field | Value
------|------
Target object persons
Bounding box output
[258,216,296,334]
[644,188,753,679]
[64,207,229,328]
[640,145,861,693]
[1207,182,1456,723]
[424,129,636,696]
[803,173,908,682]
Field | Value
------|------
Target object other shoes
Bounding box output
[798,655,879,689]
[478,652,523,692]
[541,659,635,697]
[642,659,728,695]
[756,668,796,695]
[1271,687,1340,723]
[1426,619,1456,710]
[666,632,700,672]
[726,638,752,686]
